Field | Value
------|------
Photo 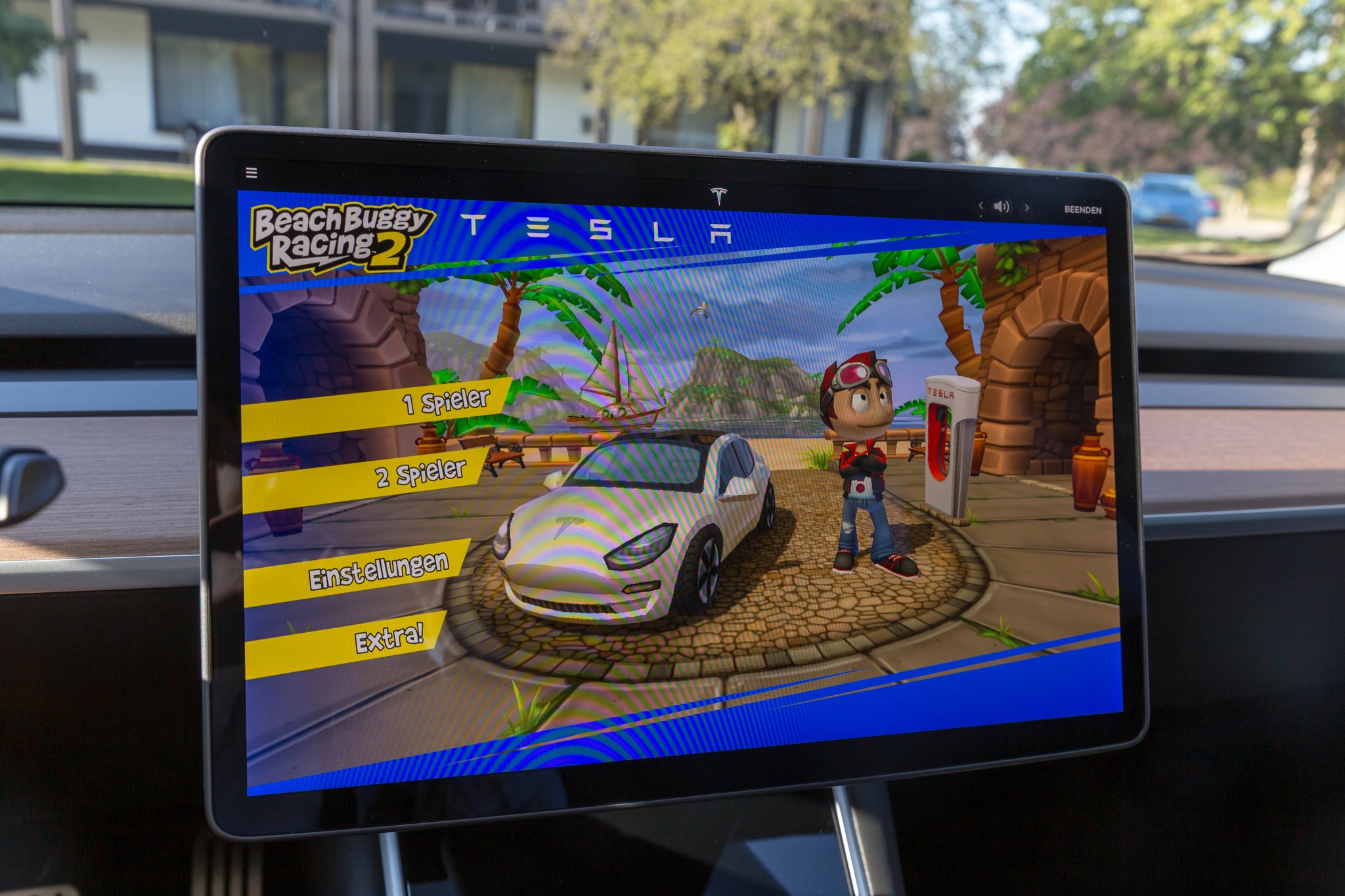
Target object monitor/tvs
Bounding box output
[382,783,869,896]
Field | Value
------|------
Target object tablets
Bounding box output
[191,125,1152,842]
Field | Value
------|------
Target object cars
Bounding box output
[1126,171,1221,232]
